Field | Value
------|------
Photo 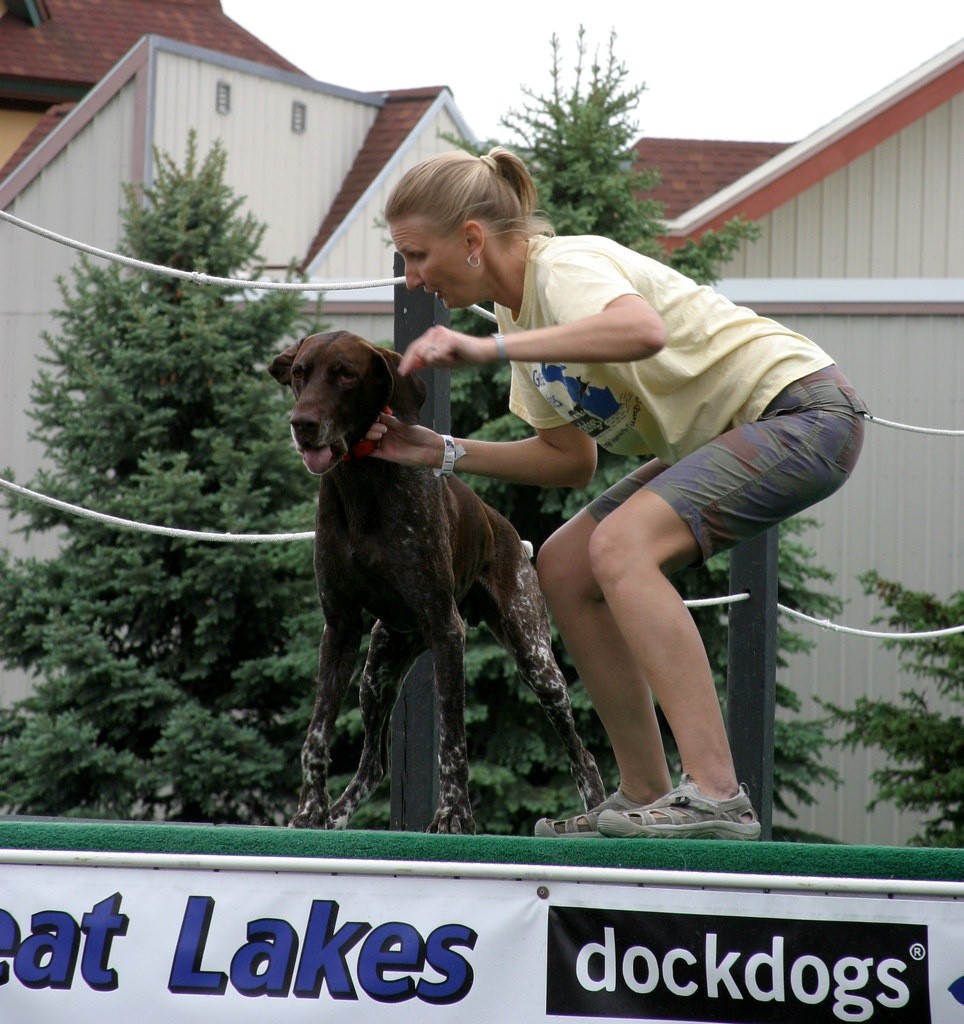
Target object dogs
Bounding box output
[266,328,608,836]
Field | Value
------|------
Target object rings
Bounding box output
[431,345,437,351]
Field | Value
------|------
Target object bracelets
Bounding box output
[491,332,508,365]
[433,432,467,477]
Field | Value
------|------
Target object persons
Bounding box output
[361,146,873,836]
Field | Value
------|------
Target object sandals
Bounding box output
[597,774,761,841]
[535,791,647,839]
